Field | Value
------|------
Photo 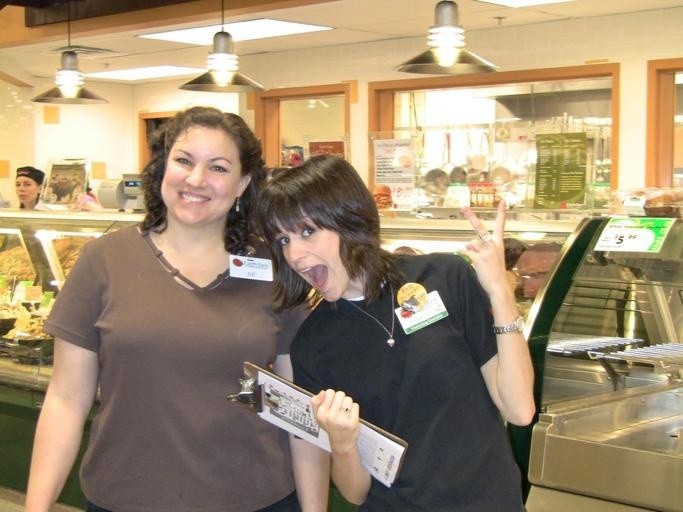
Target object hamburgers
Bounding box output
[374,186,391,209]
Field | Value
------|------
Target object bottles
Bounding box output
[445,181,499,207]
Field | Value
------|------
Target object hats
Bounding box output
[16,166,45,185]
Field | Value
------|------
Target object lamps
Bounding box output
[176,0,268,95]
[28,0,109,103]
[394,1,504,76]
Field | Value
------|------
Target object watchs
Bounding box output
[491,315,524,336]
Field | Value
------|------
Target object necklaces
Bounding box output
[339,271,397,349]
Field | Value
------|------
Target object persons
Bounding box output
[23,106,299,512]
[253,155,537,511]
[15,166,53,211]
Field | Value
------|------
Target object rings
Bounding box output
[477,231,492,242]
[343,407,350,414]
